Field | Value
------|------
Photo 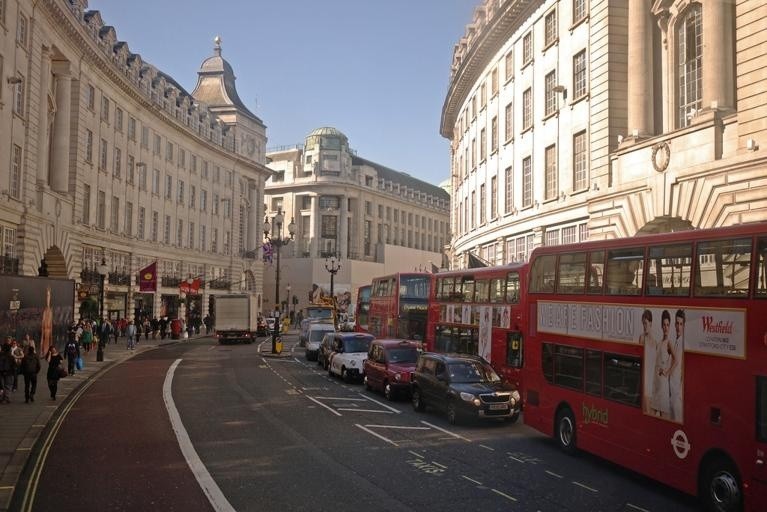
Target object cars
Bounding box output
[264,315,284,334]
[299,294,522,430]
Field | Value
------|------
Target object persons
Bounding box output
[293,309,303,328]
[0,313,214,403]
[638,310,656,415]
[654,309,675,419]
[669,309,683,421]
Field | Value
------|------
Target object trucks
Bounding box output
[213,291,262,345]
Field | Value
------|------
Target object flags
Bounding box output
[139,262,158,292]
[262,242,273,264]
[178,277,200,295]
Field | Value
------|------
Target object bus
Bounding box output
[522,221,767,512]
[426,262,600,407]
[355,284,371,333]
[369,271,431,343]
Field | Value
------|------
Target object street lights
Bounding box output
[262,205,298,356]
[324,253,343,298]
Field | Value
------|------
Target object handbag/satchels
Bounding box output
[57,367,66,378]
[75,357,83,370]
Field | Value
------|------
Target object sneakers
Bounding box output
[0,385,56,403]
